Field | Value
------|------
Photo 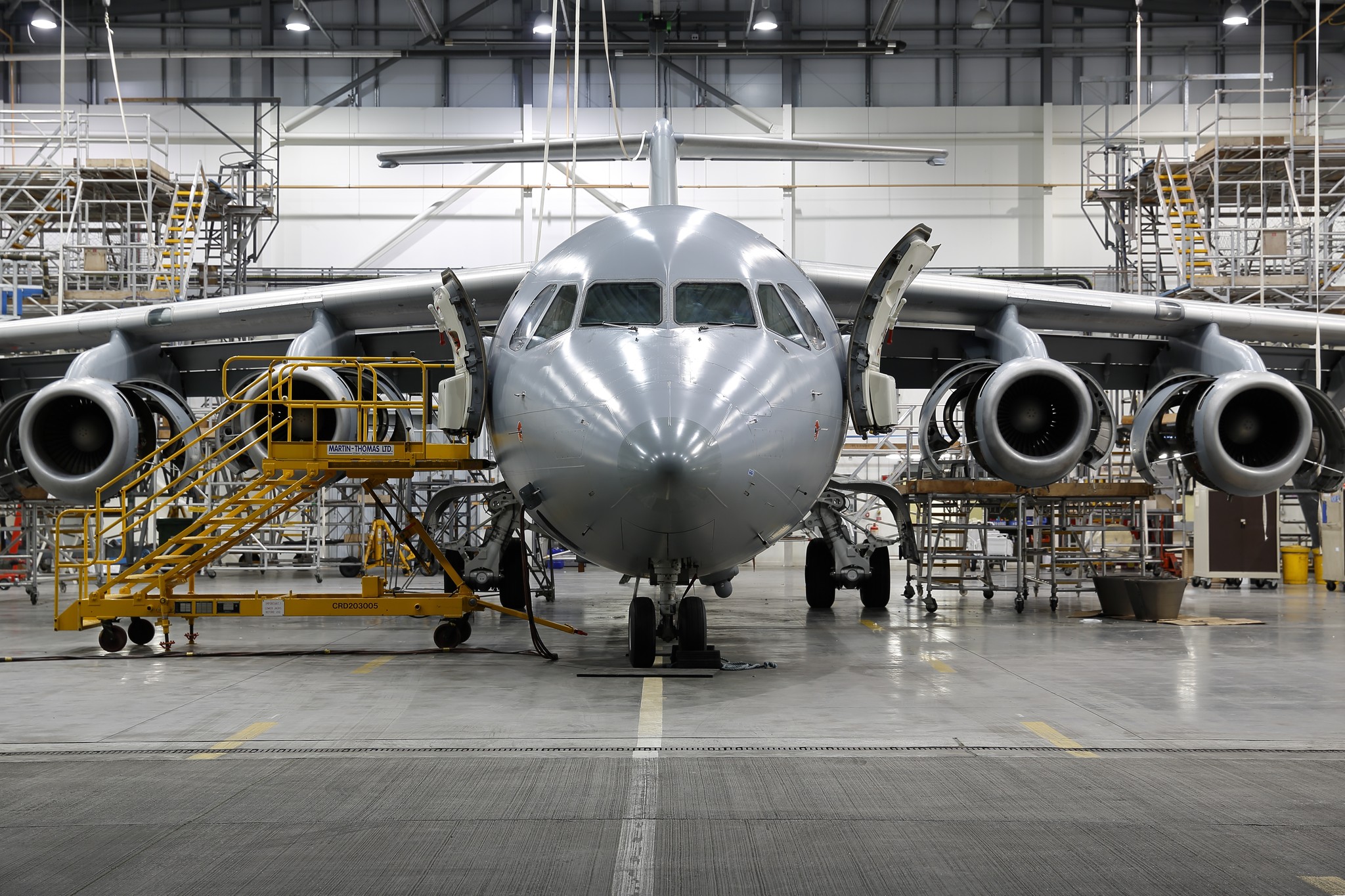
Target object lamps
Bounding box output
[753,0,778,30]
[1223,0,1250,25]
[533,0,557,35]
[30,0,57,29]
[285,0,311,31]
[971,0,994,30]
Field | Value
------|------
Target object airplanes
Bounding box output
[0,119,1345,673]
